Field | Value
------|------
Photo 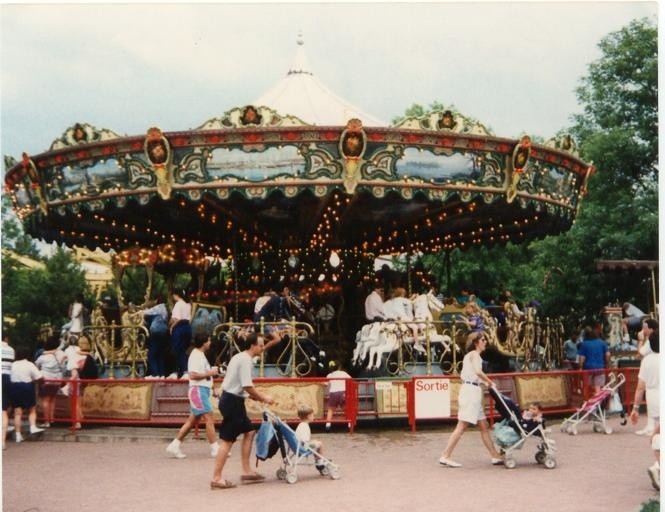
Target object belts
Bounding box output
[462,380,479,387]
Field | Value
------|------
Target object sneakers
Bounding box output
[144,372,189,379]
[635,428,655,435]
[2,423,50,450]
[210,448,231,458]
[166,447,186,458]
[61,385,69,396]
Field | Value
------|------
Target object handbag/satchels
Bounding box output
[607,393,622,415]
[44,373,63,386]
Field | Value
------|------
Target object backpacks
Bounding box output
[82,355,99,383]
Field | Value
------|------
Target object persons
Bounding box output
[522,402,542,419]
[296,407,332,470]
[366,282,541,353]
[122,288,192,379]
[67,293,112,333]
[620,298,662,490]
[440,332,504,468]
[166,332,231,458]
[324,361,352,432]
[211,334,275,487]
[1,331,90,450]
[253,281,306,369]
[562,325,612,419]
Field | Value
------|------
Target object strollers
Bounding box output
[561,372,626,434]
[479,377,558,468]
[248,399,342,483]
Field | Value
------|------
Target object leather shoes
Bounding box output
[241,472,266,484]
[211,480,236,490]
[439,457,461,467]
[491,458,504,465]
[648,466,660,491]
